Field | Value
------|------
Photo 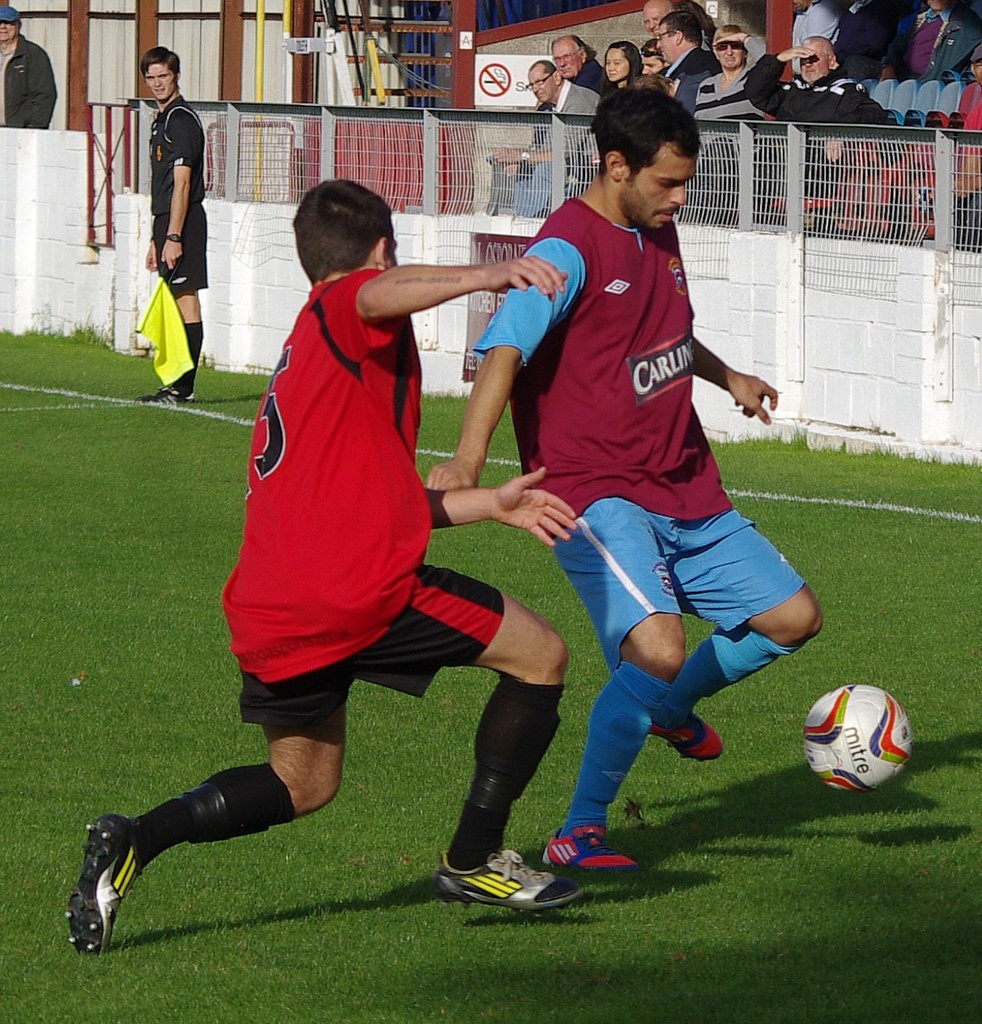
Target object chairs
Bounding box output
[849,79,966,117]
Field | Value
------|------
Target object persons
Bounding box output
[136,47,208,407]
[492,0,982,253]
[0,5,57,130]
[66,179,584,955]
[423,90,824,881]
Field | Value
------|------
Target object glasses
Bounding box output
[800,54,832,65]
[526,68,556,90]
[712,42,744,50]
[641,49,664,57]
[656,30,676,40]
[552,48,580,62]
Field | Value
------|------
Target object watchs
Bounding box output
[522,149,530,161]
[166,234,183,244]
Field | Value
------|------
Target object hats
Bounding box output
[0,7,20,21]
[970,43,982,62]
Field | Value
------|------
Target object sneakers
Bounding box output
[136,387,195,406]
[67,815,141,957]
[541,826,640,872]
[435,850,594,917]
[648,716,724,760]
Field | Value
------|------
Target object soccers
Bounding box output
[803,684,912,790]
